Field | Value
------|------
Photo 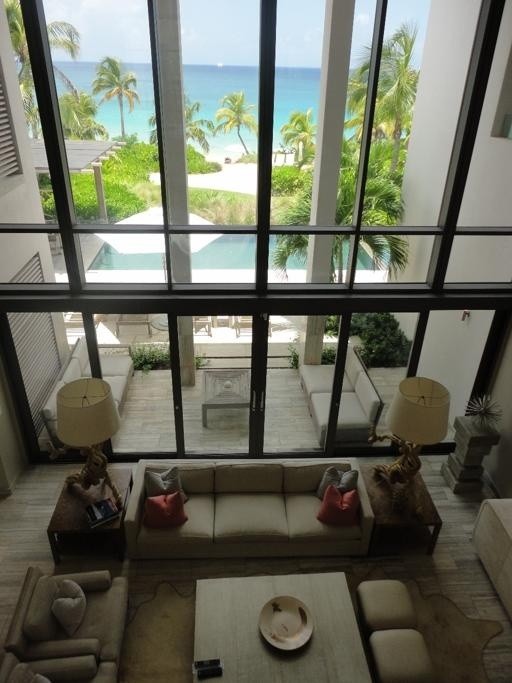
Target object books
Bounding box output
[85,498,120,529]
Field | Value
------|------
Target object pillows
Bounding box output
[314,466,358,501]
[143,490,188,529]
[144,466,189,503]
[6,662,50,683]
[50,578,88,636]
[315,484,360,525]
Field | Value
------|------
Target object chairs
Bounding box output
[3,567,130,661]
[0,652,118,683]
[64,311,273,339]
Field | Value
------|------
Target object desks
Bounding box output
[470,498,511,622]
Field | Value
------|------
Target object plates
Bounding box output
[258,595,314,652]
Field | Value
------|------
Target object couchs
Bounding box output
[39,337,135,450]
[123,457,377,560]
[299,347,385,446]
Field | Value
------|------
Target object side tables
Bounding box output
[46,467,134,564]
[360,464,442,556]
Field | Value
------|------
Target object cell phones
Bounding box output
[197,666,223,680]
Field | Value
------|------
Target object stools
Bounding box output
[369,628,437,683]
[356,579,417,630]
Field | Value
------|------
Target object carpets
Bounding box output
[118,564,503,683]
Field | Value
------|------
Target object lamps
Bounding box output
[55,377,122,512]
[367,377,451,521]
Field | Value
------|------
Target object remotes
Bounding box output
[194,659,221,669]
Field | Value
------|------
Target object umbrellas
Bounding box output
[94,206,222,283]
